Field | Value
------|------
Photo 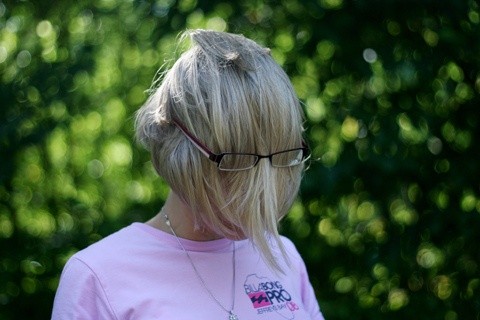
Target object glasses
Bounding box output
[171,117,312,171]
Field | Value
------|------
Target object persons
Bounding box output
[59,27,323,320]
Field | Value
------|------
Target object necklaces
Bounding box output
[160,206,240,320]
